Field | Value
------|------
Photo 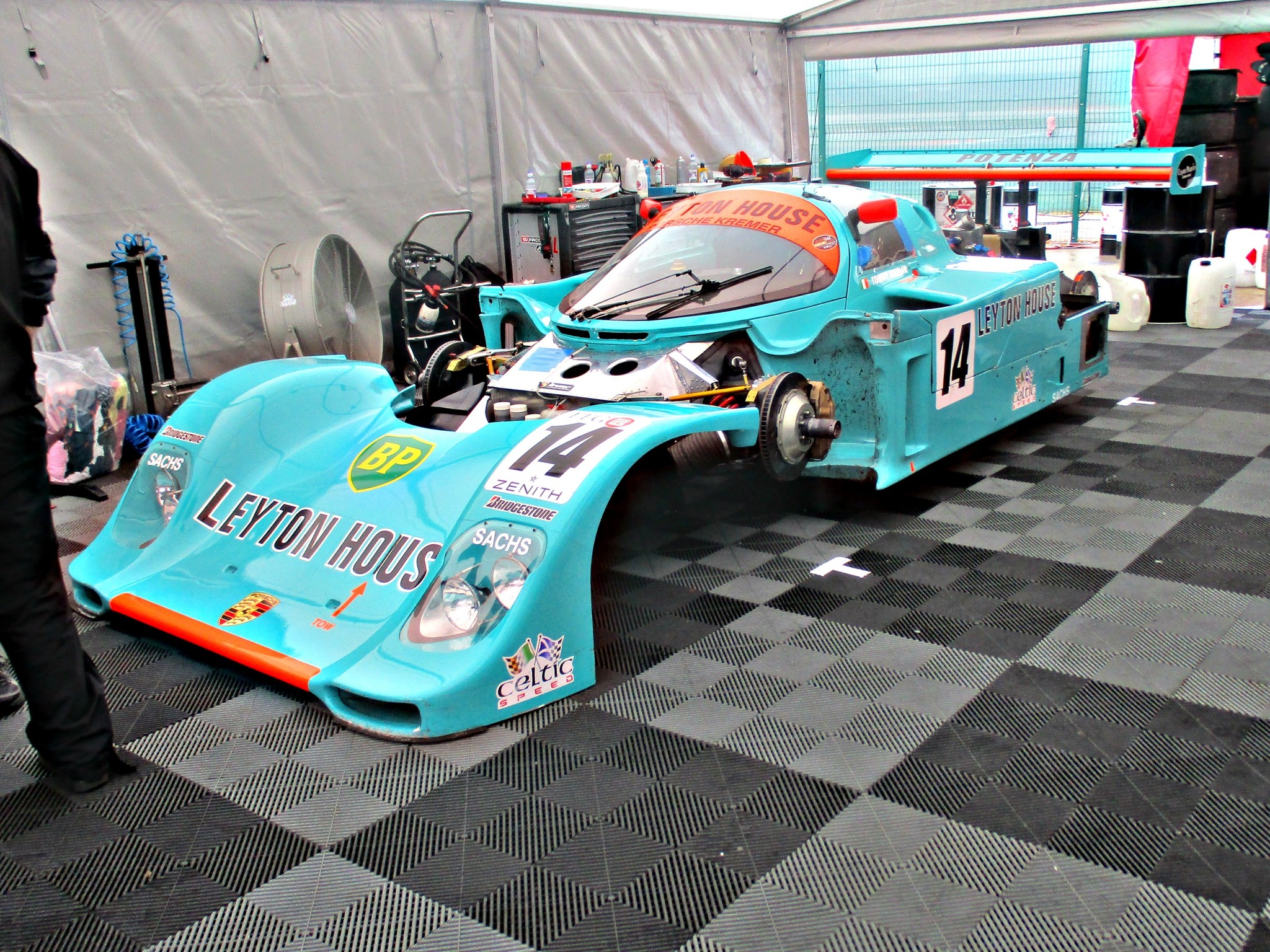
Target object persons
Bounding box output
[1,139,116,795]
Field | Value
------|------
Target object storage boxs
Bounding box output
[648,185,676,196]
[677,182,722,194]
[561,161,573,192]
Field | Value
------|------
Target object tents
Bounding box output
[2,0,1270,396]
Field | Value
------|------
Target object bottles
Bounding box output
[624,158,640,192]
[585,162,594,183]
[636,160,648,199]
[561,161,573,198]
[697,163,708,183]
[643,159,651,189]
[954,214,975,231]
[677,156,686,184]
[595,161,603,176]
[525,171,536,198]
[601,167,613,182]
[654,159,666,188]
[688,155,698,183]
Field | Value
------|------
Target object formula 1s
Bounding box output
[71,143,1212,746]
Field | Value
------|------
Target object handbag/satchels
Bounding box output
[30,347,131,482]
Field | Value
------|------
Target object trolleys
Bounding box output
[389,209,491,387]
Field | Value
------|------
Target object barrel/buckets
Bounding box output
[1092,268,1150,332]
[1185,256,1236,329]
[1100,189,1123,258]
[1224,228,1269,290]
[1001,187,1038,230]
[1120,182,1216,327]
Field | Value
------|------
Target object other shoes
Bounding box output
[22,724,107,793]
[0,667,26,712]
